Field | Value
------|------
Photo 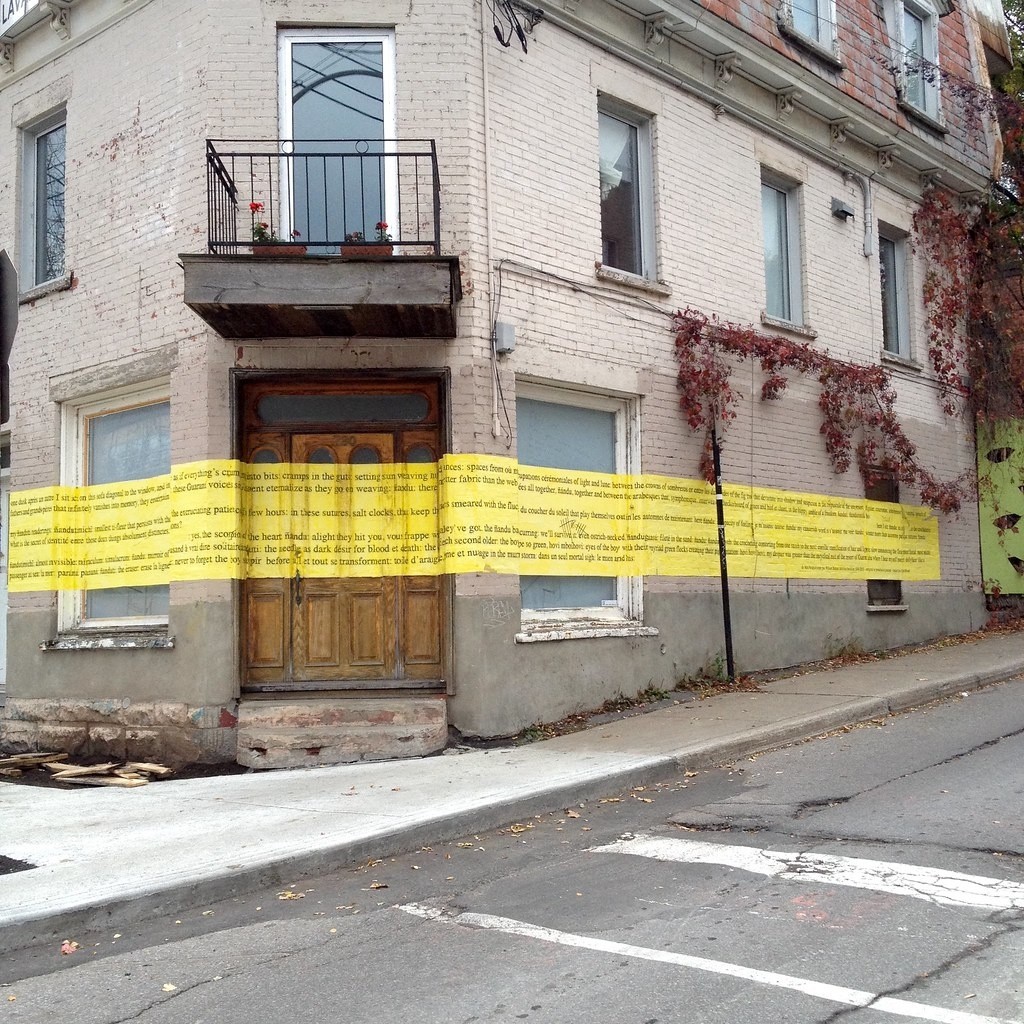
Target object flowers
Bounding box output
[249,202,300,243]
[345,222,393,244]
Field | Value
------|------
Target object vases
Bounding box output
[251,245,307,255]
[340,246,394,256]
